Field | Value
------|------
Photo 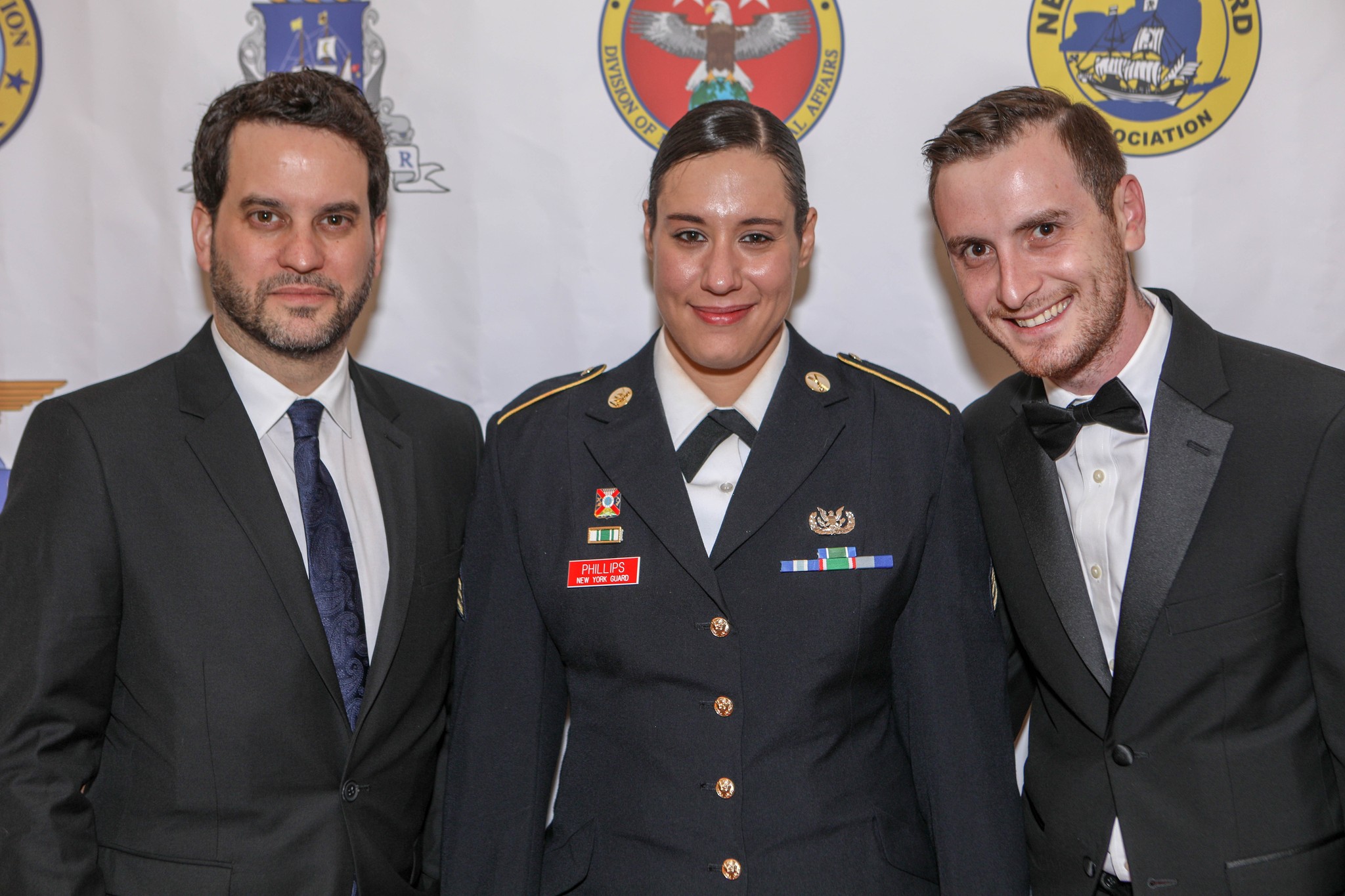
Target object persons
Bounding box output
[920,86,1345,896]
[0,70,485,896]
[440,99,1036,896]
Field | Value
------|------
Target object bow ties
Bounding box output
[1021,377,1151,461]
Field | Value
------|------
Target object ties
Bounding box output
[286,397,372,730]
